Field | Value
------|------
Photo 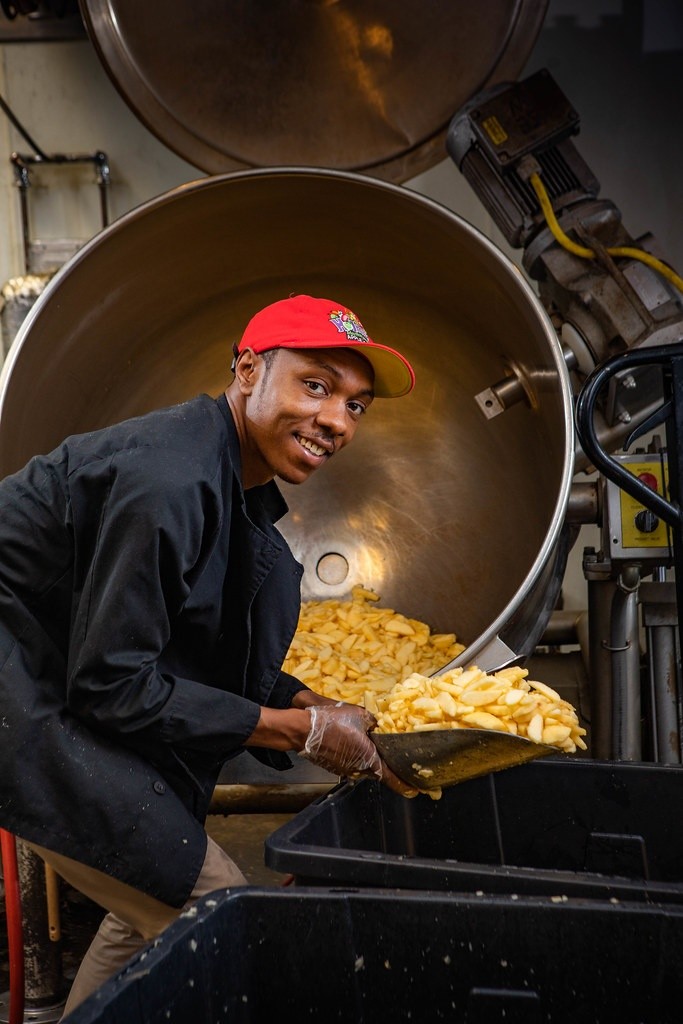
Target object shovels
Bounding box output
[369,728,558,794]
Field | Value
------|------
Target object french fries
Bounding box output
[279,584,466,708]
[361,664,587,753]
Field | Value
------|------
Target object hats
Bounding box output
[230,294,416,399]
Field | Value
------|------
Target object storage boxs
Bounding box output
[262,754,683,905]
[58,886,683,1024]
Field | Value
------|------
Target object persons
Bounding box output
[0,294,415,1024]
